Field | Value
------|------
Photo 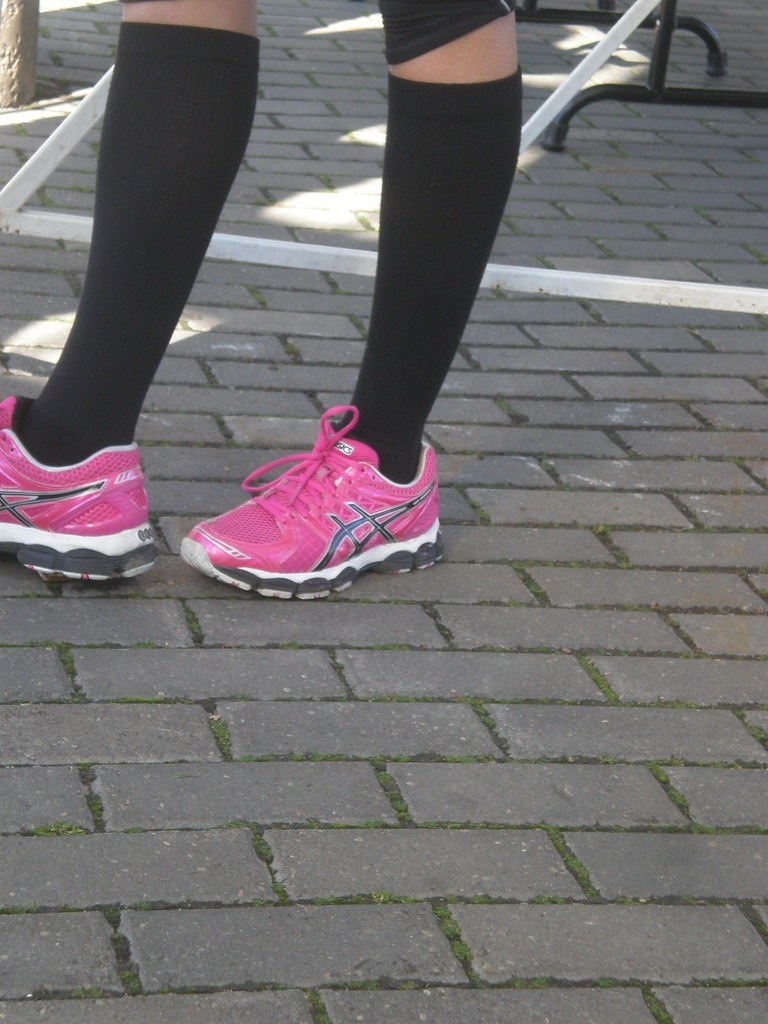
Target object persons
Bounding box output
[1,0,521,600]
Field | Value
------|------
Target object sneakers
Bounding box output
[181,406,442,599]
[0,396,159,580]
